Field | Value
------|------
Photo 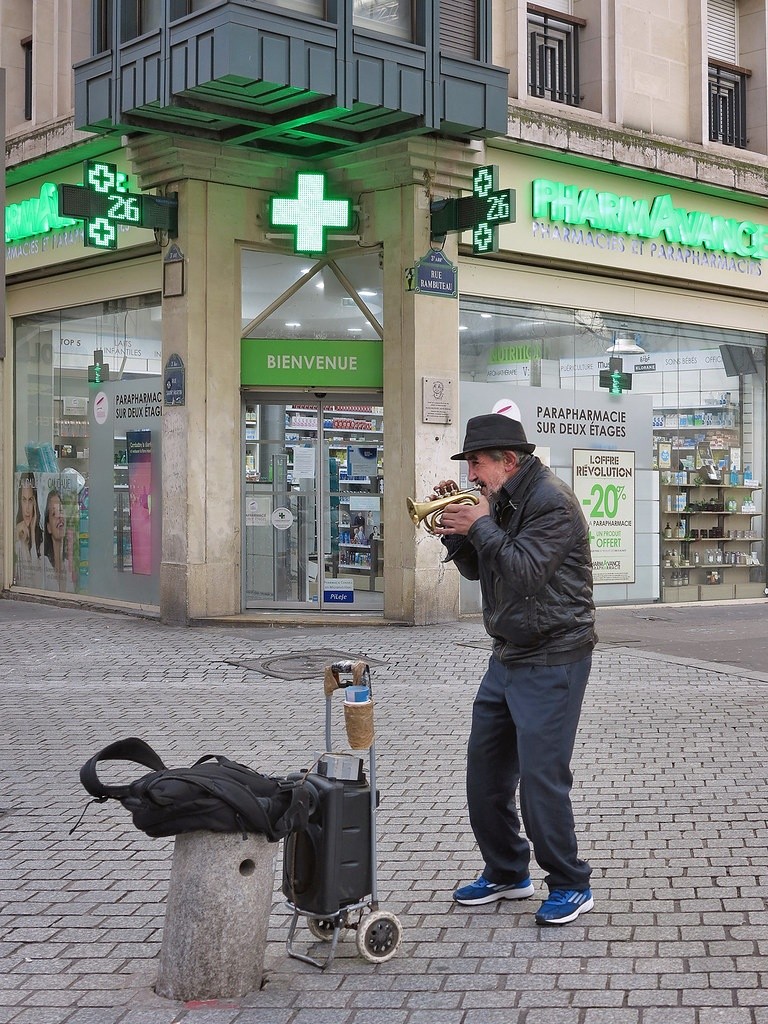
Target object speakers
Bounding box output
[280,769,380,913]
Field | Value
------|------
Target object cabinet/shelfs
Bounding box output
[245,405,383,592]
[54,394,89,477]
[114,436,133,567]
[651,401,768,602]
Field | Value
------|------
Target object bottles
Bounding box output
[694,548,723,564]
[670,572,689,587]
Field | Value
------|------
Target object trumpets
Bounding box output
[405,482,482,538]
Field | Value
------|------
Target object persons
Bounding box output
[12,472,79,594]
[425,415,597,923]
[352,511,380,542]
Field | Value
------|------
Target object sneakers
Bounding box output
[535,890,594,923]
[453,875,534,905]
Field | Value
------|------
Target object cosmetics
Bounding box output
[337,514,373,568]
[659,463,760,589]
[52,421,130,467]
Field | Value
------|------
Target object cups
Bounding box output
[345,686,370,703]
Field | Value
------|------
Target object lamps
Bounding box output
[607,328,645,354]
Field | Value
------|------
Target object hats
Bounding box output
[451,414,536,460]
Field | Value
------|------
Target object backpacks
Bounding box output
[68,737,310,842]
